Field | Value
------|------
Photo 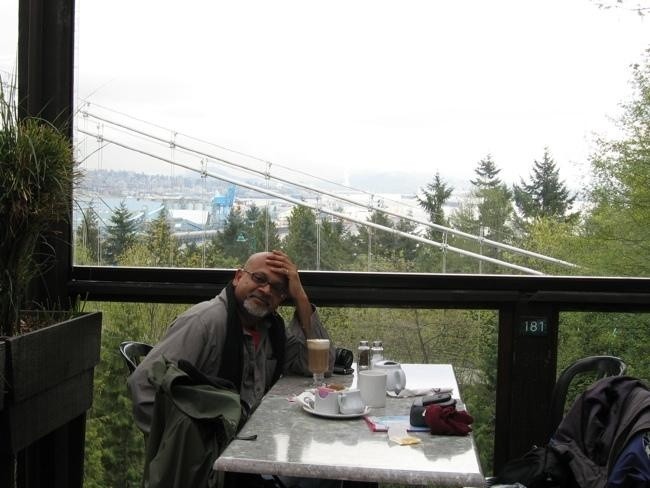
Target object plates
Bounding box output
[301,403,371,419]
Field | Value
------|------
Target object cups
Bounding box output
[358,371,387,407]
[314,388,338,412]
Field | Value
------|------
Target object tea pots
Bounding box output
[374,360,405,392]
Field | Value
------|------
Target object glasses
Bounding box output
[243,270,283,296]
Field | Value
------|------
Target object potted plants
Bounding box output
[0,72,105,453]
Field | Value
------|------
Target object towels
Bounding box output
[424,402,474,437]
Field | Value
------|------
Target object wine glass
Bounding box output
[307,339,330,387]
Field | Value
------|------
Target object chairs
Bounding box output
[544,353,628,448]
[503,376,650,487]
[119,339,154,376]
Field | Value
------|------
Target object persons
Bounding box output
[125,251,337,488]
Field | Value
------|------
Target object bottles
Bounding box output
[357,340,370,371]
[371,340,383,369]
[340,390,363,413]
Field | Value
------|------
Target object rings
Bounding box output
[286,268,290,275]
